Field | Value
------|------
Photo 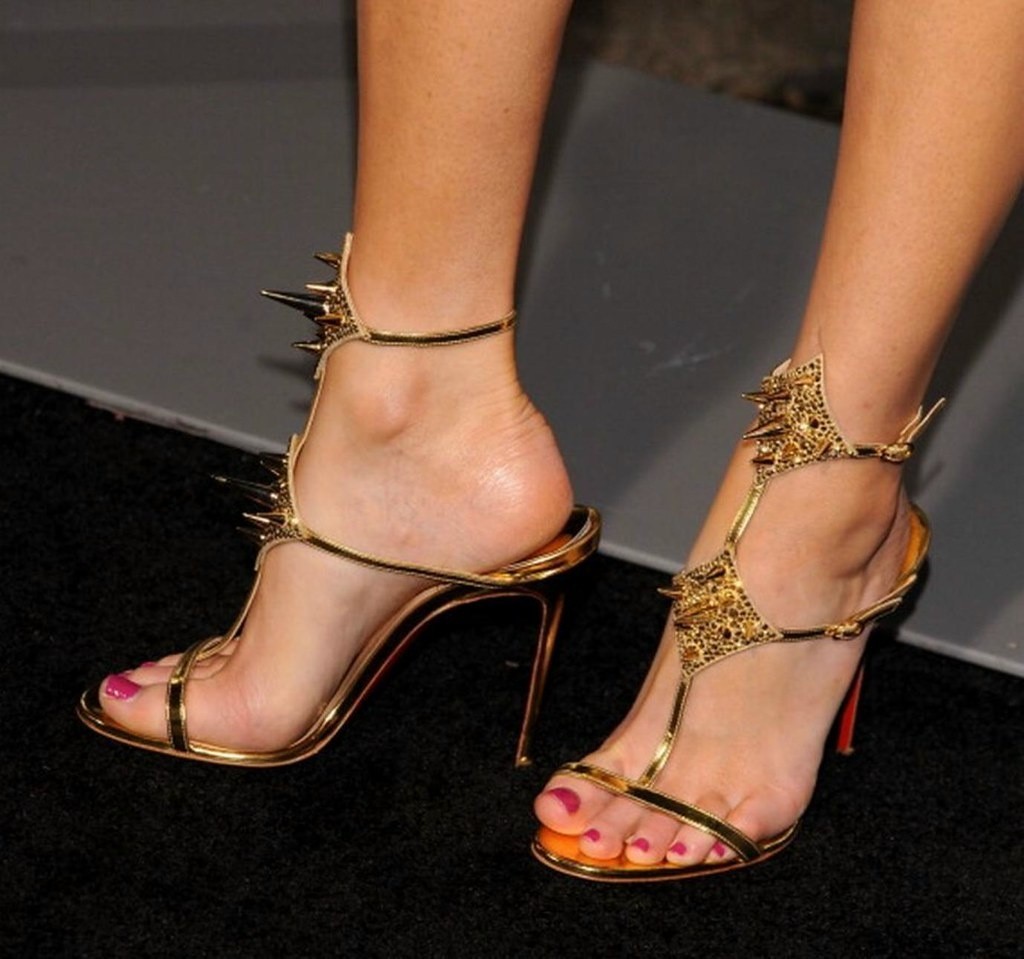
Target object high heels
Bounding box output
[530,352,948,884]
[72,231,600,768]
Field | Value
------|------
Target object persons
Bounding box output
[77,0,1024,885]
